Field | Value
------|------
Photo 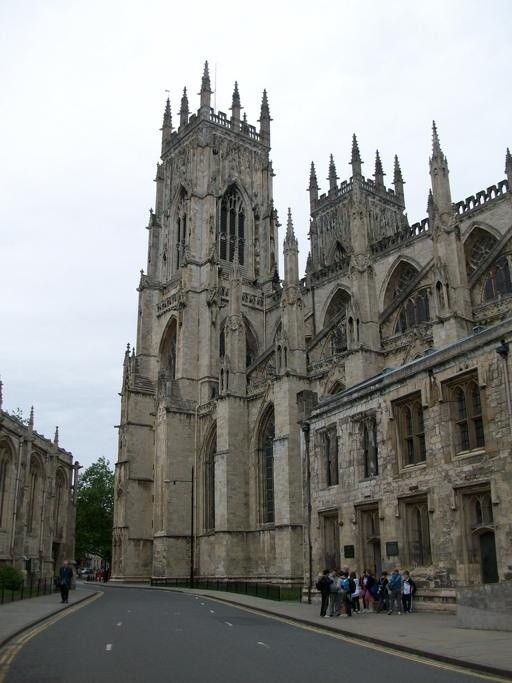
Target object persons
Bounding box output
[93,563,110,583]
[57,559,74,604]
[315,566,415,617]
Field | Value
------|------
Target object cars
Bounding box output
[78,566,91,573]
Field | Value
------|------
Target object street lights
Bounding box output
[290,418,312,597]
